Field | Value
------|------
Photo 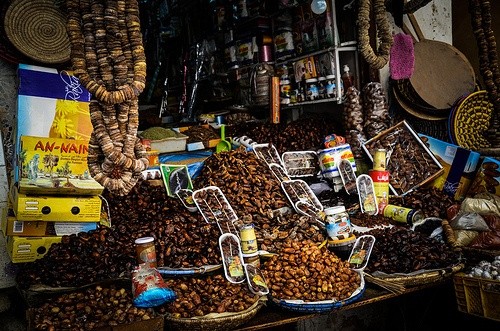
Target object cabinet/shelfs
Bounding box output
[174,0,360,109]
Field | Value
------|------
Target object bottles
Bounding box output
[305,64,354,100]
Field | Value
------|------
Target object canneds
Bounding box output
[384,204,424,224]
[374,148,386,171]
[135,236,158,268]
[305,74,337,101]
[369,169,389,215]
[324,205,352,240]
[239,224,261,277]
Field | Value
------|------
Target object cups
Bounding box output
[261,45,271,62]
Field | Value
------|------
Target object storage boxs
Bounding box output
[4,134,104,263]
[362,122,500,199]
[454,274,500,322]
[232,24,255,39]
[137,130,189,154]
